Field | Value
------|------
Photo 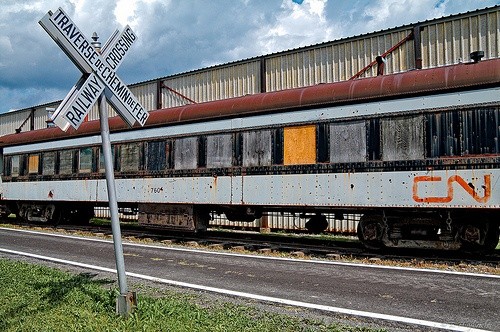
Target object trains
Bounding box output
[0,50,500,259]
